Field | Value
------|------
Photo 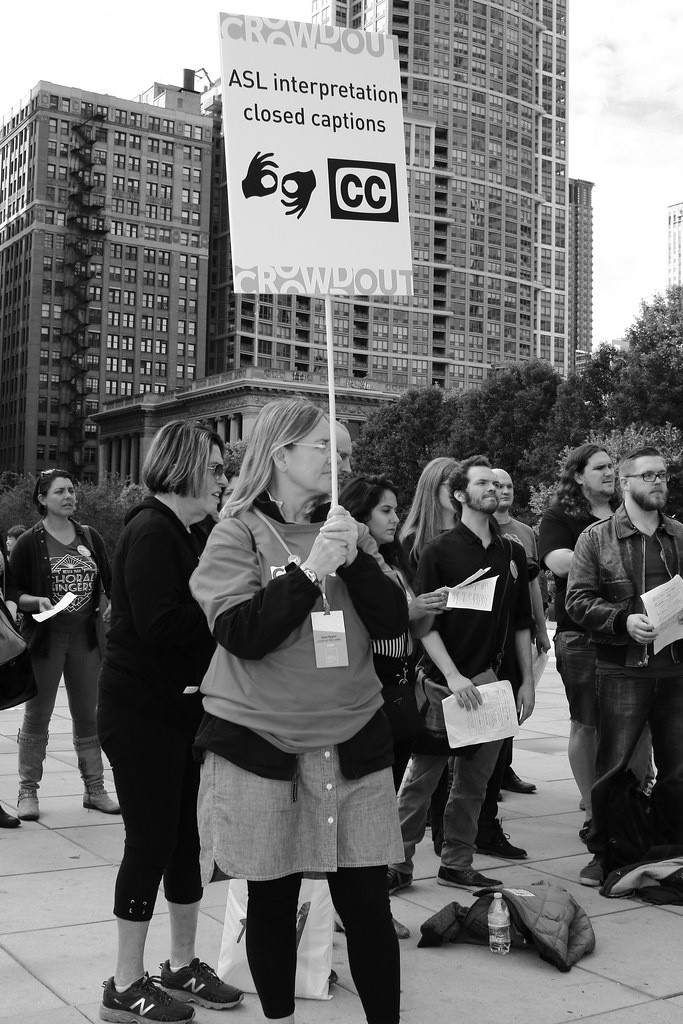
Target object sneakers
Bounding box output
[473,830,528,859]
[388,867,414,896]
[159,958,245,1011]
[579,854,603,885]
[437,865,504,892]
[98,970,195,1024]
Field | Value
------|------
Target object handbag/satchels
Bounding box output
[81,525,110,657]
[216,878,334,1001]
[414,664,498,742]
[381,683,425,747]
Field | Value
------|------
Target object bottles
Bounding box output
[487,892,510,954]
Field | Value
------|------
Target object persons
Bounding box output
[564,446,682,888]
[188,396,410,1024]
[1,440,683,986]
[8,467,123,819]
[94,419,246,1024]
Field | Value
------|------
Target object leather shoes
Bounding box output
[501,769,537,791]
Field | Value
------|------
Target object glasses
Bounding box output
[173,463,226,477]
[623,471,671,482]
[38,469,68,494]
[284,441,329,452]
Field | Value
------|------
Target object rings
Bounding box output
[344,515,348,521]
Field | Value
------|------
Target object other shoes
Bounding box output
[332,907,412,939]
[0,803,21,828]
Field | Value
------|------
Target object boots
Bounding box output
[72,734,120,814]
[16,728,49,820]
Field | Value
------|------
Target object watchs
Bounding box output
[298,564,320,589]
